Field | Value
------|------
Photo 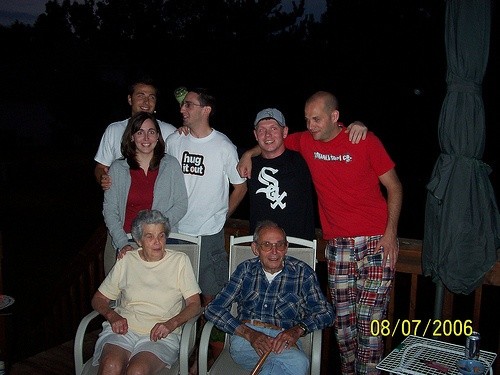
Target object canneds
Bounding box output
[465,331,480,360]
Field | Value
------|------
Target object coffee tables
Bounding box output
[376,335,496,375]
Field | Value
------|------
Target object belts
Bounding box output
[242,320,282,330]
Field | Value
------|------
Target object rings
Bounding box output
[284,340,289,343]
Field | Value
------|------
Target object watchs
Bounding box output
[295,323,306,337]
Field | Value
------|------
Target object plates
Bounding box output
[0,293,15,312]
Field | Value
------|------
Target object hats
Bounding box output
[254,108,285,129]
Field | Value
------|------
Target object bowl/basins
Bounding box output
[456,360,485,375]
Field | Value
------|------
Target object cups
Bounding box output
[464,332,480,359]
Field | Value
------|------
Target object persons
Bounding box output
[165,89,247,350]
[205,221,335,375]
[104,111,188,306]
[95,82,190,190]
[92,209,202,375]
[237,93,402,375]
[247,108,316,248]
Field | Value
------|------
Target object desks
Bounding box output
[315,227,500,356]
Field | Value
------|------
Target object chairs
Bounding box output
[74,234,323,375]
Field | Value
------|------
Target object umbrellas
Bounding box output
[422,0,500,341]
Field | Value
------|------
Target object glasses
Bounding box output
[180,101,206,108]
[132,94,156,102]
[255,241,289,252]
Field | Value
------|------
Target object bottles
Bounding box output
[173,86,188,105]
